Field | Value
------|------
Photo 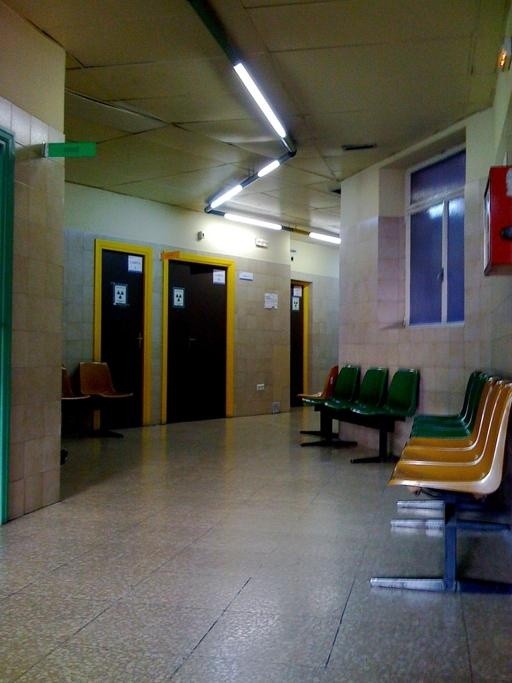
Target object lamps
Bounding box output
[188,0,341,244]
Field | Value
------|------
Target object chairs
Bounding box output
[62,362,134,439]
[298,367,422,467]
[368,366,512,593]
